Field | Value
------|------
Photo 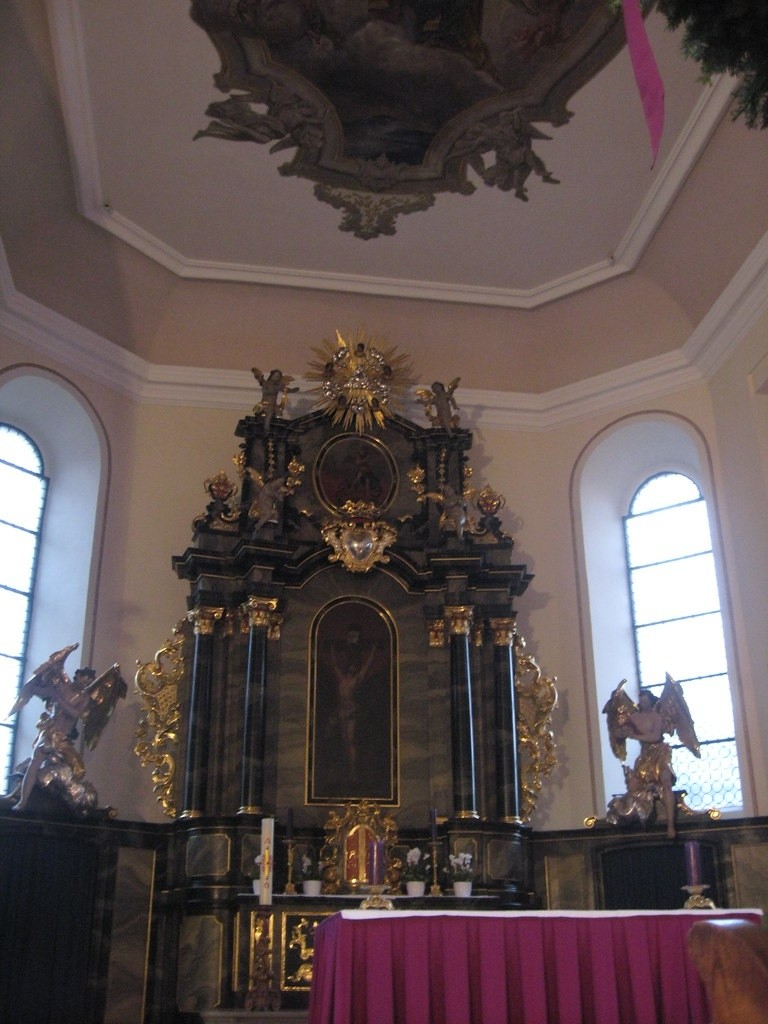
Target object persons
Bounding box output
[13,667,96,811]
[331,645,376,776]
[614,689,677,839]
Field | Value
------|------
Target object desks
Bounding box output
[308,908,764,1024]
[234,893,502,1012]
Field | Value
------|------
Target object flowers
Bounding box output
[397,843,432,879]
[301,852,325,880]
[446,847,478,881]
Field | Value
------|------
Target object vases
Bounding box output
[302,879,322,895]
[454,881,472,896]
[405,881,427,895]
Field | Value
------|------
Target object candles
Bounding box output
[684,835,702,885]
[369,841,381,883]
[286,807,295,840]
[429,804,438,843]
[259,817,274,905]
